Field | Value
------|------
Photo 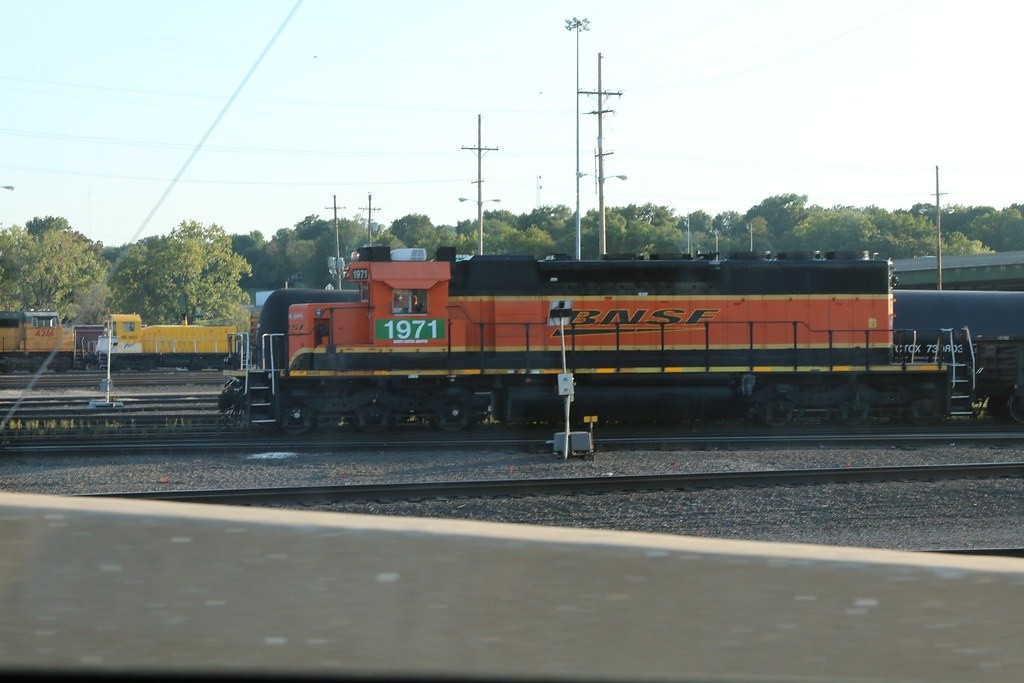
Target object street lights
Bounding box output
[563,16,590,258]
[599,175,627,258]
[459,198,501,255]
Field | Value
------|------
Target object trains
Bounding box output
[0,310,258,374]
[218,247,1024,427]
